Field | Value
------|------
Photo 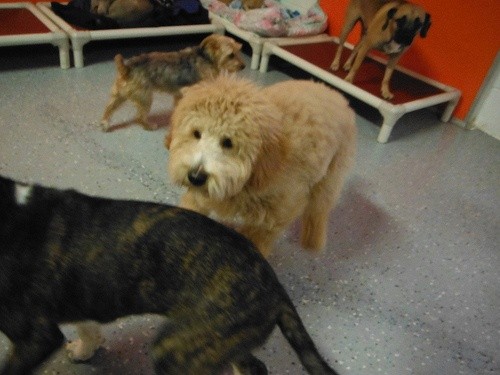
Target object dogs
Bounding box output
[90,0,155,25]
[219,0,267,11]
[329,0,432,101]
[164,68,357,262]
[101,34,247,132]
[0,174,340,375]
